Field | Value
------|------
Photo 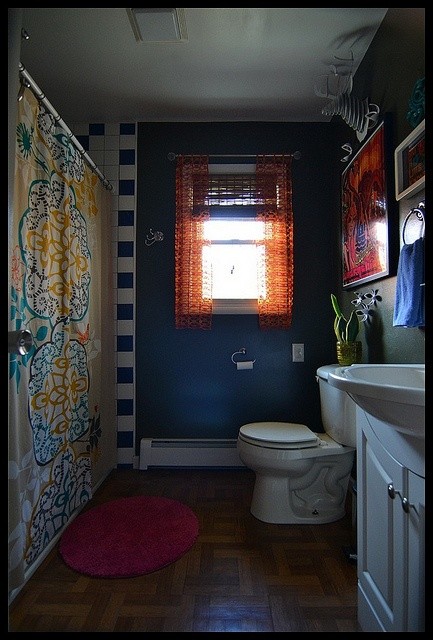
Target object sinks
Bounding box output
[333,364,425,393]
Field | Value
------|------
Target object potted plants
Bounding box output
[329,295,362,366]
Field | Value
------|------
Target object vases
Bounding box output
[364,323,379,363]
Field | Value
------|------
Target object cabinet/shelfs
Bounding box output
[356,432,424,633]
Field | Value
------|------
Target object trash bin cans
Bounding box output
[343,481,357,564]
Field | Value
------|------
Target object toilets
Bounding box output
[235,362,357,526]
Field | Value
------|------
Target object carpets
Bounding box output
[58,497,200,580]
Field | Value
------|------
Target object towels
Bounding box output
[391,237,423,328]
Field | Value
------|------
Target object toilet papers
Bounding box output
[235,361,254,370]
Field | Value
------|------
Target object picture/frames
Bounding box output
[339,121,396,289]
[395,119,425,202]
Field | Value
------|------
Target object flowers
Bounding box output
[351,290,382,322]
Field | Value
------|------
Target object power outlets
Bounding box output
[292,344,303,363]
